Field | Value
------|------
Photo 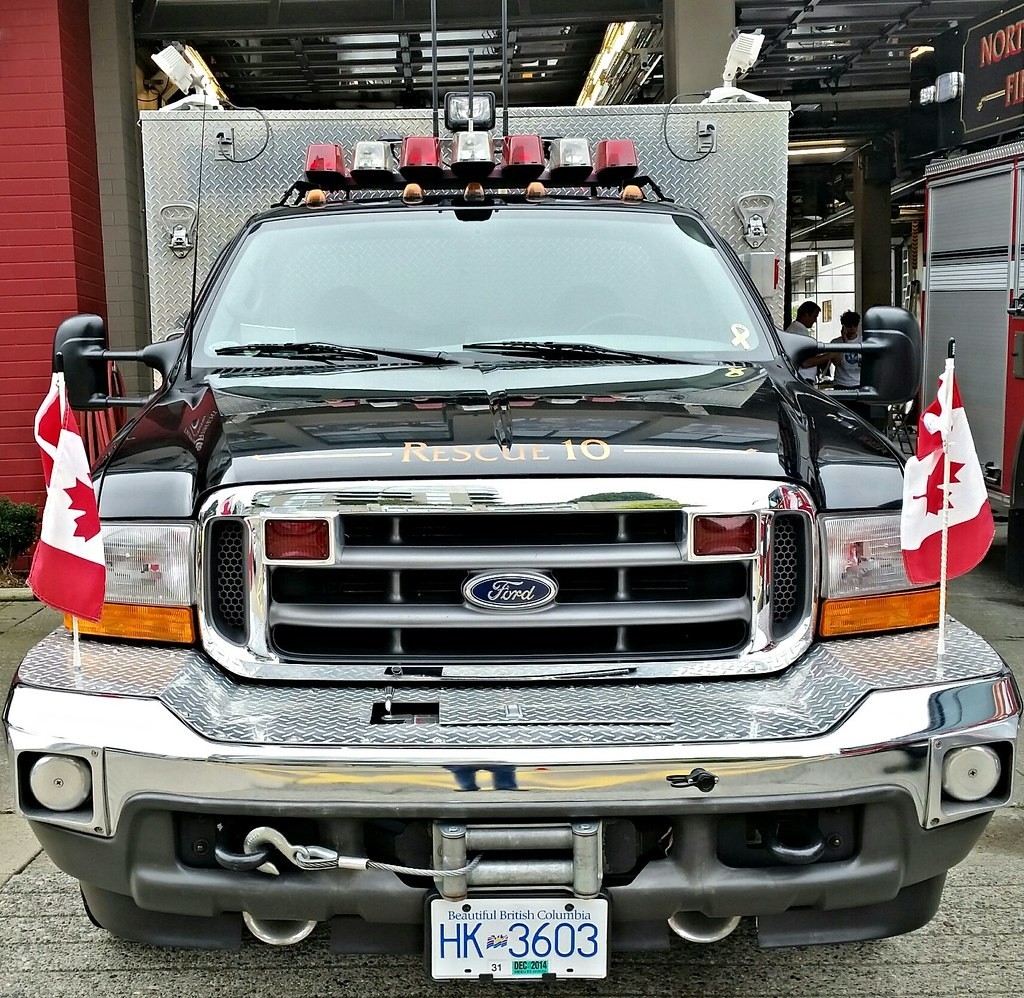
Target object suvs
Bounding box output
[3,0,1023,998]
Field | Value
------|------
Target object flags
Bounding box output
[30,379,104,623]
[899,368,994,585]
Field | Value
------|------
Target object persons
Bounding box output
[830,310,862,390]
[785,300,822,385]
